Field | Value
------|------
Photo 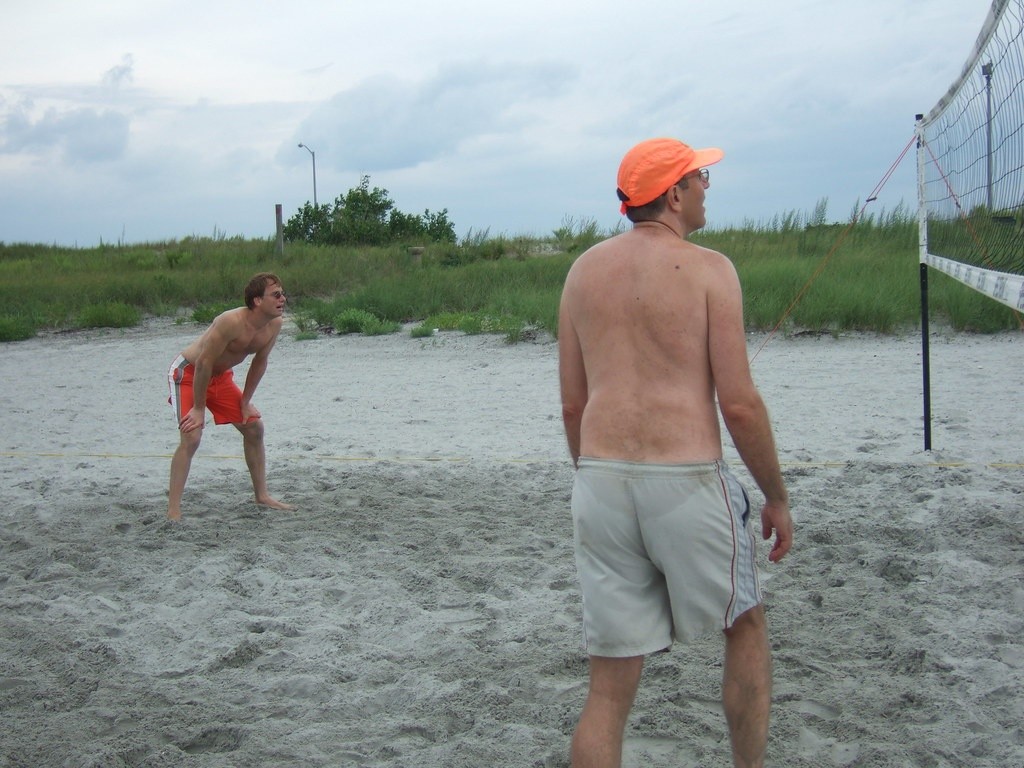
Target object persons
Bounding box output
[167,272,292,519]
[558,137,793,768]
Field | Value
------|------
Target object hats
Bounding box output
[617,139,724,215]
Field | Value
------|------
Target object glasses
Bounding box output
[263,290,286,299]
[661,169,710,196]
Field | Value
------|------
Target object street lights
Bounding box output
[298,143,318,226]
